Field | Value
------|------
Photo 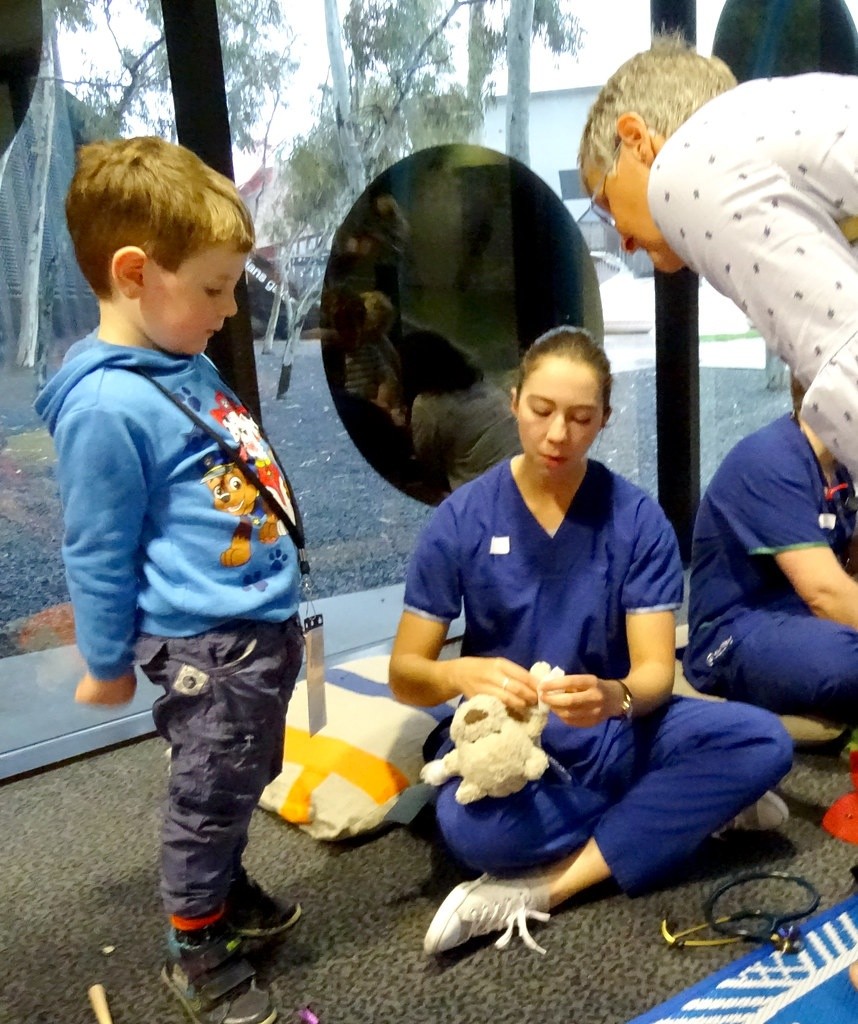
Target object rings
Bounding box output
[502,677,511,689]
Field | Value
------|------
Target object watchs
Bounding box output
[611,679,634,721]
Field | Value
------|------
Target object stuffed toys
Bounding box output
[418,661,569,805]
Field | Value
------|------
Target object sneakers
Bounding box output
[224,880,302,937]
[711,790,789,838]
[424,873,550,956]
[160,928,278,1024]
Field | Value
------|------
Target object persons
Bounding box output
[683,374,858,725]
[580,46,858,485]
[388,326,795,954]
[32,135,305,1024]
[319,172,525,495]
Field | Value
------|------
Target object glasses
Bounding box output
[591,138,624,229]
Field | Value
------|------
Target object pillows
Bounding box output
[257,655,463,842]
